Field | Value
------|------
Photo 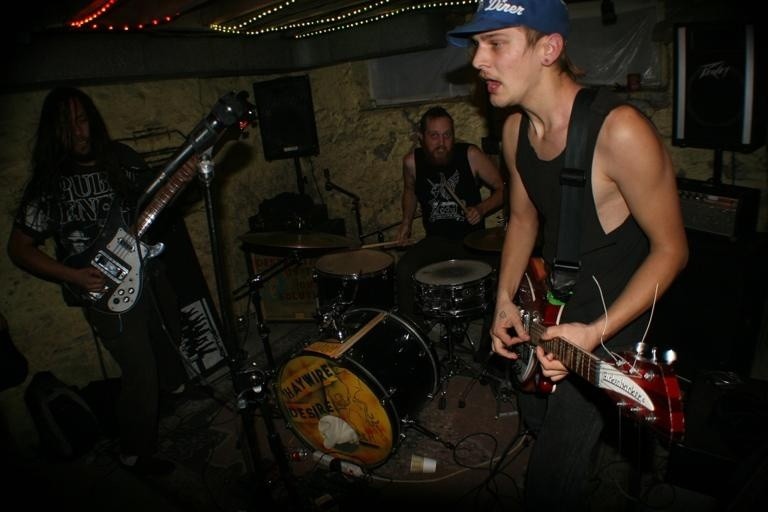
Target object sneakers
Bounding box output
[119,454,177,481]
[423,318,448,343]
[172,384,214,402]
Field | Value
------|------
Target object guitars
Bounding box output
[60,89,256,314]
[511,256,685,452]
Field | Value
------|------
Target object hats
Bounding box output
[447,0,569,47]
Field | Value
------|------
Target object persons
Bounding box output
[395,105,504,261]
[445,0,689,512]
[7,86,214,485]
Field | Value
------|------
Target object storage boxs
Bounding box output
[244,218,346,329]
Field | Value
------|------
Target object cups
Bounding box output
[410,454,436,475]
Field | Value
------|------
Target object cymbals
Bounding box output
[238,231,361,250]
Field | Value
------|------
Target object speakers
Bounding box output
[672,20,764,154]
[252,73,321,163]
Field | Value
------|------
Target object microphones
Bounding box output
[137,95,244,206]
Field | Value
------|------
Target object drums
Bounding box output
[275,308,440,469]
[410,258,496,320]
[314,250,396,312]
